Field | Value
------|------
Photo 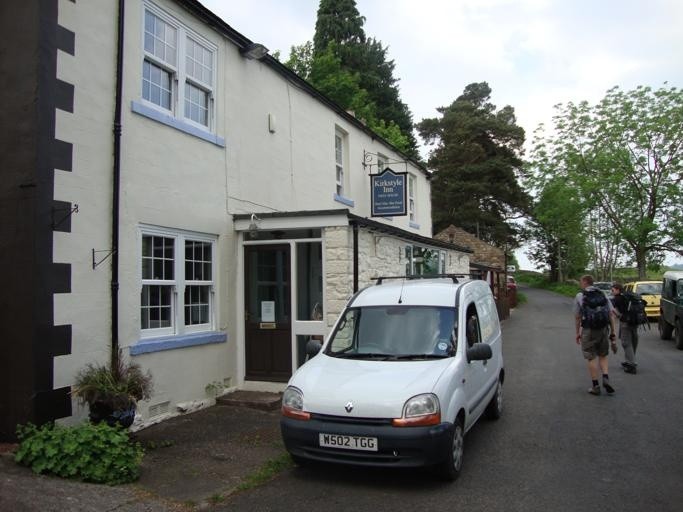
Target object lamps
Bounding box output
[240,42,270,61]
[249,212,262,238]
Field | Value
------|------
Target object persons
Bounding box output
[573,275,619,395]
[610,284,639,373]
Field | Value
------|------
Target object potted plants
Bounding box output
[64,345,154,430]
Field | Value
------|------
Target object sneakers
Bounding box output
[603,383,615,393]
[588,385,601,395]
[624,367,637,374]
[621,360,637,367]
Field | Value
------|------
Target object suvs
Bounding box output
[659,271,683,350]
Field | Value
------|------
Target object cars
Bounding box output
[504,275,517,307]
[506,266,516,276]
[593,281,616,308]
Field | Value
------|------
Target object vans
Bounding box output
[281,268,506,482]
[623,281,663,318]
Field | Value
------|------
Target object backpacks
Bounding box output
[576,289,610,330]
[620,292,647,325]
